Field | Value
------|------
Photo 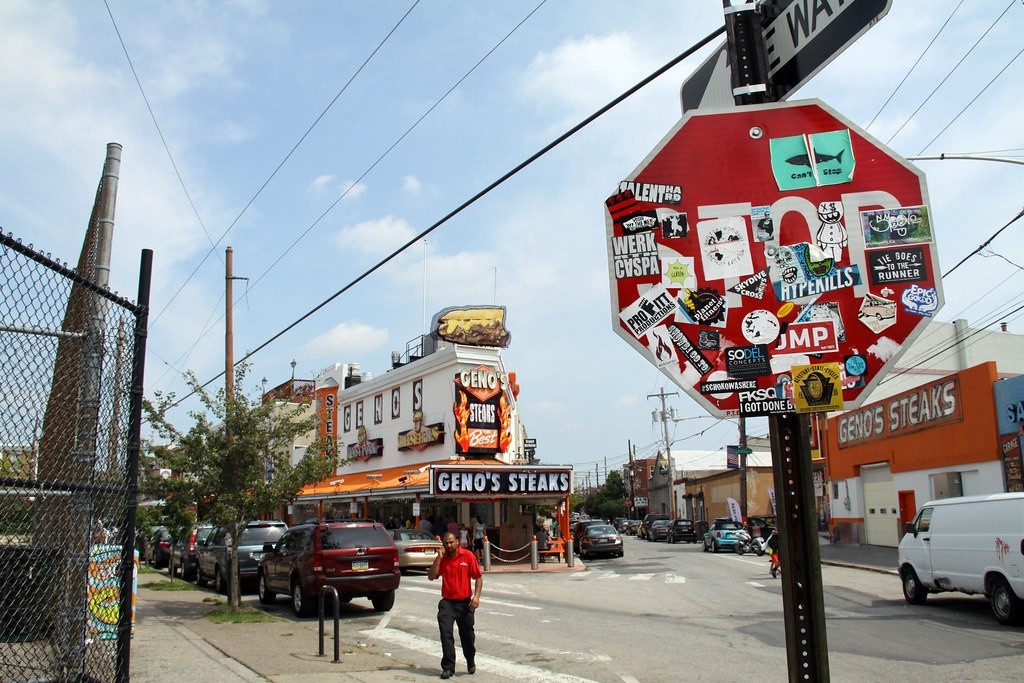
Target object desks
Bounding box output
[547,541,559,546]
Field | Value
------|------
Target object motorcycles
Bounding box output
[733,524,765,557]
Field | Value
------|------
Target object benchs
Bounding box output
[537,537,565,562]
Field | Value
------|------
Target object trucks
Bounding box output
[897,492,1024,626]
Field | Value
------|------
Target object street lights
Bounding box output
[628,469,636,520]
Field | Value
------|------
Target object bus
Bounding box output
[572,512,579,515]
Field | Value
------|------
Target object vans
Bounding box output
[637,513,670,540]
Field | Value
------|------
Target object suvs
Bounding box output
[139,525,216,581]
[666,519,698,544]
[256,518,400,621]
[716,518,776,540]
[570,518,606,553]
[193,519,288,595]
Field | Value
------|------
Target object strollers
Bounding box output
[764,529,782,577]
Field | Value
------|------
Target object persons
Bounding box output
[385,515,487,561]
[90,519,118,546]
[534,527,553,564]
[427,531,483,678]
[771,530,781,579]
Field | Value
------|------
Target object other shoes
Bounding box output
[441,670,453,679]
[466,658,476,675]
[770,567,777,579]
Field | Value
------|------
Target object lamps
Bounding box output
[328,456,465,492]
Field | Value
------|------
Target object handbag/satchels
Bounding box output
[481,538,484,544]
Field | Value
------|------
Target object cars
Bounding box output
[578,525,624,560]
[569,515,589,530]
[648,520,673,542]
[703,522,743,553]
[387,529,443,575]
[614,518,642,536]
